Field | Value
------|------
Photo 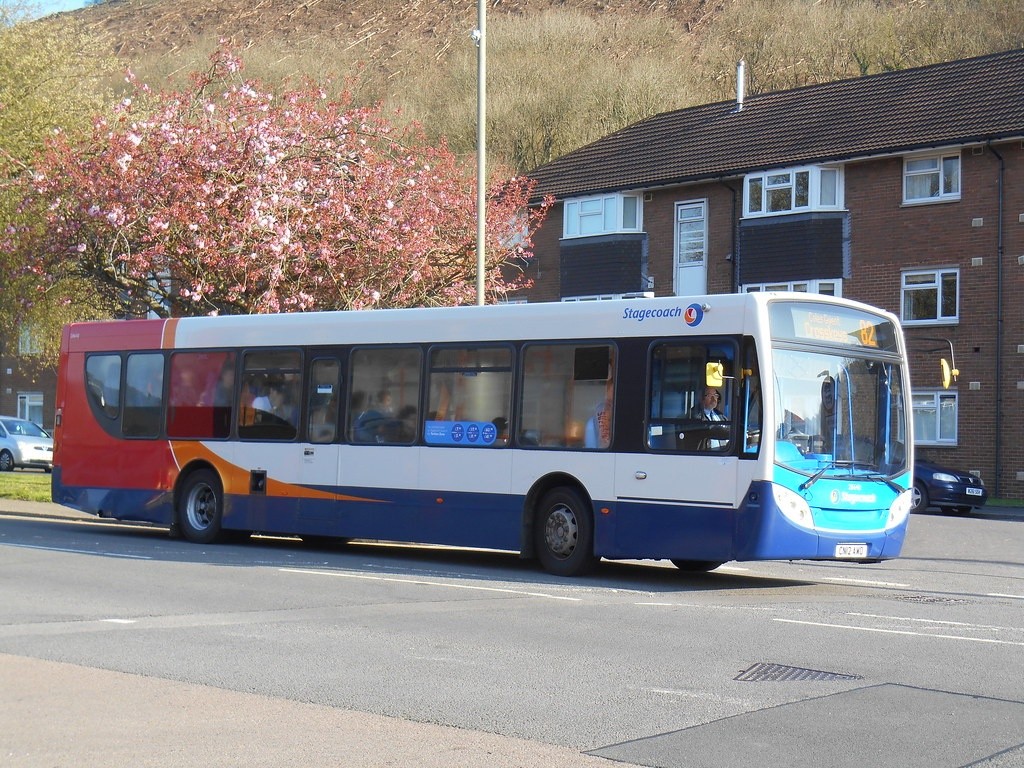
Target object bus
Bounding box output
[47,290,963,578]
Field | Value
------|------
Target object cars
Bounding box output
[0,414,53,473]
[836,432,989,518]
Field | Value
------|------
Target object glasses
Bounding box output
[703,393,720,399]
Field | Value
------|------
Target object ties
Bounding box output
[710,410,727,450]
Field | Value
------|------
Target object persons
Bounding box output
[682,386,749,452]
[210,369,444,443]
[491,417,507,439]
[584,403,610,447]
